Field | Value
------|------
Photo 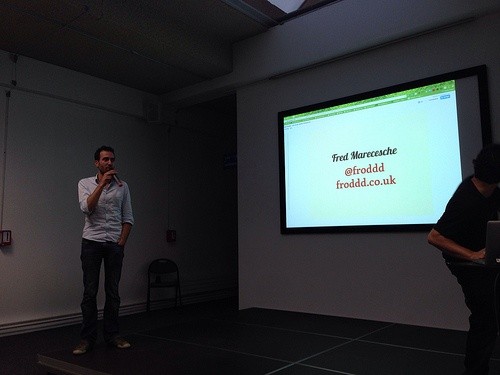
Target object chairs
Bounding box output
[146,259,180,312]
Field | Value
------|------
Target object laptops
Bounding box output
[484,221,500,266]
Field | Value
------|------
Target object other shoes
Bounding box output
[102,335,131,348]
[73,339,97,354]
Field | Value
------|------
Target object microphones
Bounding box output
[110,170,122,187]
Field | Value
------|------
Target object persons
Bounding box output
[73,146,134,354]
[428,143,500,375]
